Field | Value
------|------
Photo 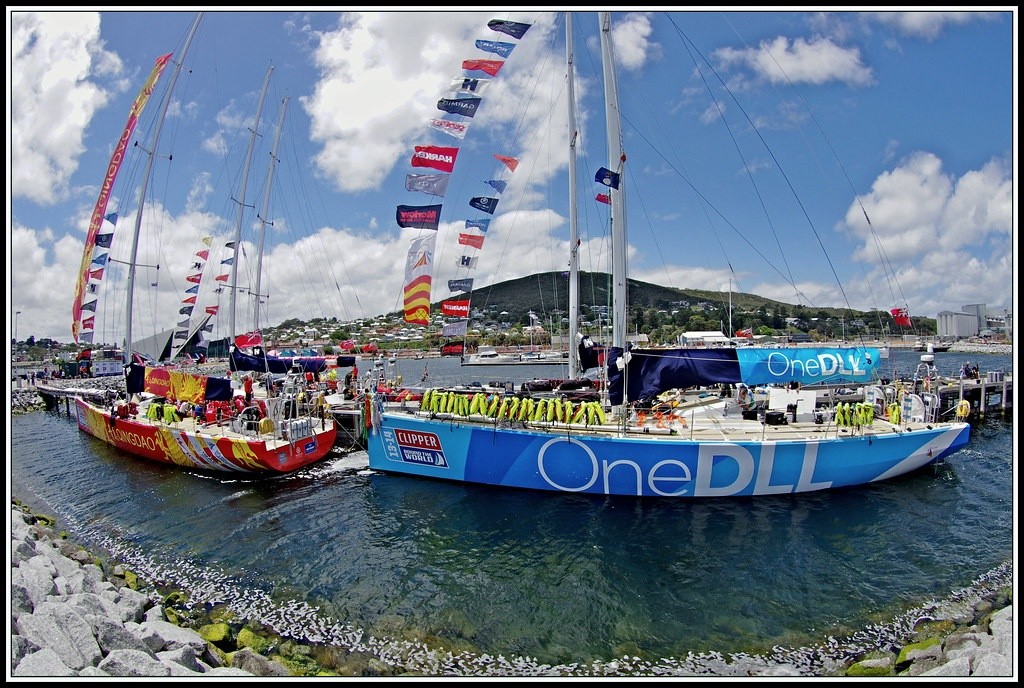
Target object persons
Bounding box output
[965,363,979,379]
[243,375,253,402]
[145,400,217,425]
[306,369,315,385]
[61,370,65,379]
[345,364,358,388]
[52,370,56,376]
[26,372,36,385]
[736,384,758,415]
[80,366,93,378]
[785,382,801,393]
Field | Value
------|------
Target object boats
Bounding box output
[469,345,502,362]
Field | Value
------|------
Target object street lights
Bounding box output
[15,310,22,369]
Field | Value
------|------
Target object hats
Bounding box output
[226,370,232,375]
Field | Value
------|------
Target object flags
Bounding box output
[206,241,235,315]
[595,167,620,190]
[362,342,378,351]
[595,193,611,204]
[235,329,262,349]
[442,153,520,338]
[78,212,119,361]
[890,308,912,326]
[395,18,532,329]
[175,237,213,341]
[338,340,355,350]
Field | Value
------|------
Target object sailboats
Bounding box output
[69,10,973,504]
